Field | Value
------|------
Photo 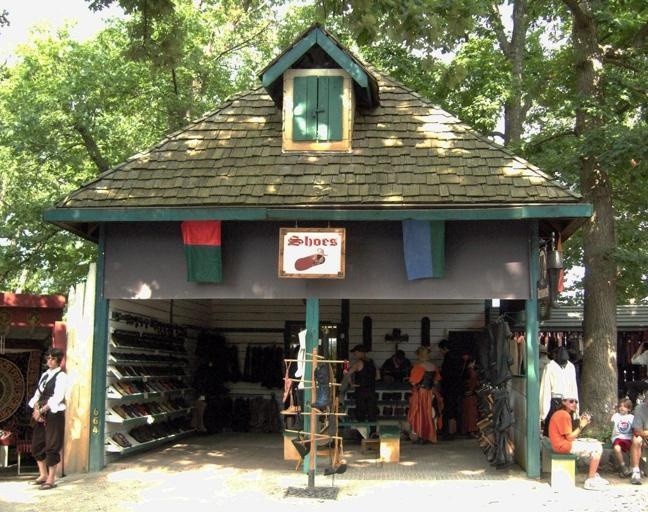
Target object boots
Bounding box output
[310,362,333,408]
[479,322,513,467]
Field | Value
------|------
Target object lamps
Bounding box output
[538,229,561,270]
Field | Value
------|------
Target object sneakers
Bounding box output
[129,396,191,417]
[129,414,195,443]
[125,365,187,376]
[120,404,135,418]
[112,432,132,448]
[118,378,188,395]
[111,382,125,396]
[115,365,128,377]
[631,468,641,485]
[620,463,631,477]
[583,472,611,491]
[279,404,302,415]
[112,405,127,419]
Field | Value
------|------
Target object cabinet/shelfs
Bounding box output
[102,328,200,457]
[337,385,438,436]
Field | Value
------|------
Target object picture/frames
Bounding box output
[277,225,347,281]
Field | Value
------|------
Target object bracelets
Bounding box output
[579,425,583,430]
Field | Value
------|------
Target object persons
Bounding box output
[28,348,70,490]
[460,354,481,439]
[630,342,647,371]
[409,347,441,445]
[549,393,610,492]
[380,350,412,385]
[540,346,581,437]
[342,345,377,439]
[611,341,648,485]
[437,340,465,441]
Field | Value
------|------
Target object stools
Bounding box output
[15,441,42,477]
[359,438,379,455]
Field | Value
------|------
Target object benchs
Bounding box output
[282,427,306,462]
[541,439,577,491]
[377,424,402,462]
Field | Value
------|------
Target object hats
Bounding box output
[350,344,371,353]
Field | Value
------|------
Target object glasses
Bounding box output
[569,400,578,404]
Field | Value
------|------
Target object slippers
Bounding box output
[28,476,50,486]
[39,480,58,490]
